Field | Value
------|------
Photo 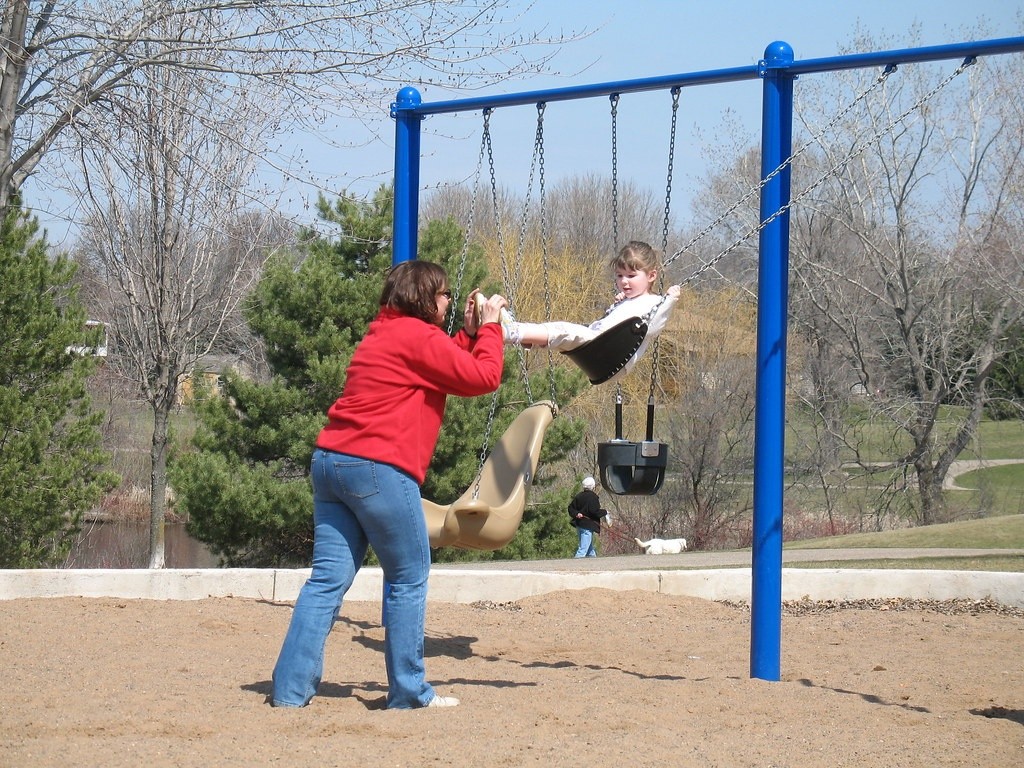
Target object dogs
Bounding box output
[635,537,687,555]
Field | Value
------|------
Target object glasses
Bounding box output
[443,289,451,299]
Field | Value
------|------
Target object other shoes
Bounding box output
[473,292,516,345]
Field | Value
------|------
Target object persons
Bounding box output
[475,241,681,381]
[271,260,508,710]
[568,476,611,558]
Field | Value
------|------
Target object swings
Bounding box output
[556,52,980,387]
[418,98,559,552]
[594,82,682,500]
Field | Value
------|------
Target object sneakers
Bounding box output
[426,691,460,709]
